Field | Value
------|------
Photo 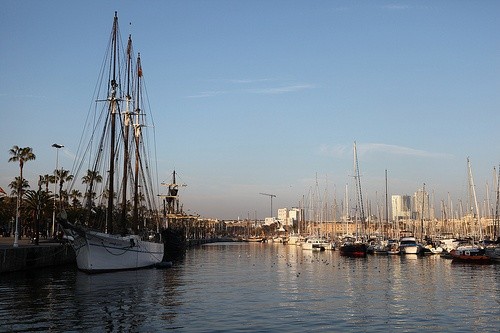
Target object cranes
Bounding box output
[257,192,277,218]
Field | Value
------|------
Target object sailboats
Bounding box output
[268,139,500,263]
[53,9,165,273]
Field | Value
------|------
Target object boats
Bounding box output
[244,238,265,241]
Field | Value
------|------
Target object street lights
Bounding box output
[51,144,64,238]
